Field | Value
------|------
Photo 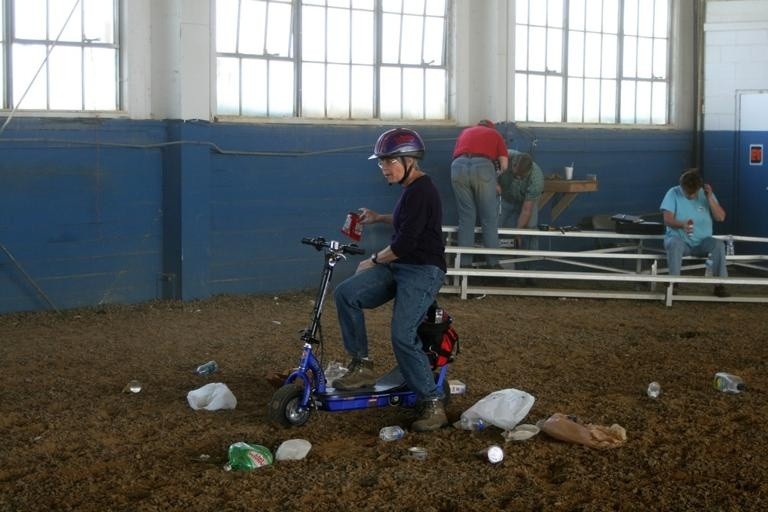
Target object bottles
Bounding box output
[648,381,660,400]
[705,253,713,278]
[688,219,695,235]
[475,446,504,464]
[714,370,747,395]
[195,360,219,376]
[460,418,485,432]
[727,235,734,256]
[379,426,405,442]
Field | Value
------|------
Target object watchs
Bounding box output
[370,251,380,265]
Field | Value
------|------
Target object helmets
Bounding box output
[367,127,426,161]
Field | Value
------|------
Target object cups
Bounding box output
[564,167,574,181]
[407,447,427,461]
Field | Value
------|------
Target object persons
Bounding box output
[495,146,546,286]
[660,168,734,297]
[330,128,453,430]
[448,117,511,269]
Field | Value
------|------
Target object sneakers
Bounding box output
[411,399,450,431]
[332,358,376,391]
[713,286,732,298]
